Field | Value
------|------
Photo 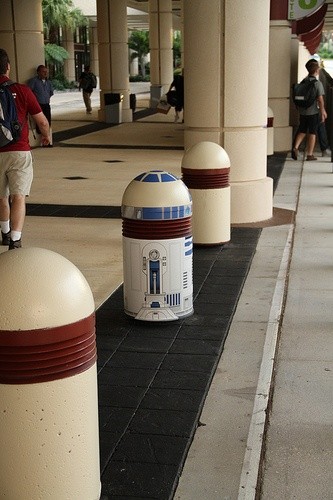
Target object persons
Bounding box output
[78,65,93,114]
[25,65,54,148]
[291,58,333,160]
[0,48,51,250]
[170,68,184,124]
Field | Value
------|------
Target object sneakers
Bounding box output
[9,238,22,250]
[1,230,11,245]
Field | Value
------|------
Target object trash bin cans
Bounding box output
[149,85,162,110]
[103,91,125,124]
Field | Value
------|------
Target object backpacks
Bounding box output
[84,71,96,89]
[293,79,318,110]
[0,80,36,149]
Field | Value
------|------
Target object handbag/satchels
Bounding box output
[157,95,171,114]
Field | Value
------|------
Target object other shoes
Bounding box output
[291,146,298,160]
[306,155,318,160]
[174,115,180,122]
[87,110,92,114]
